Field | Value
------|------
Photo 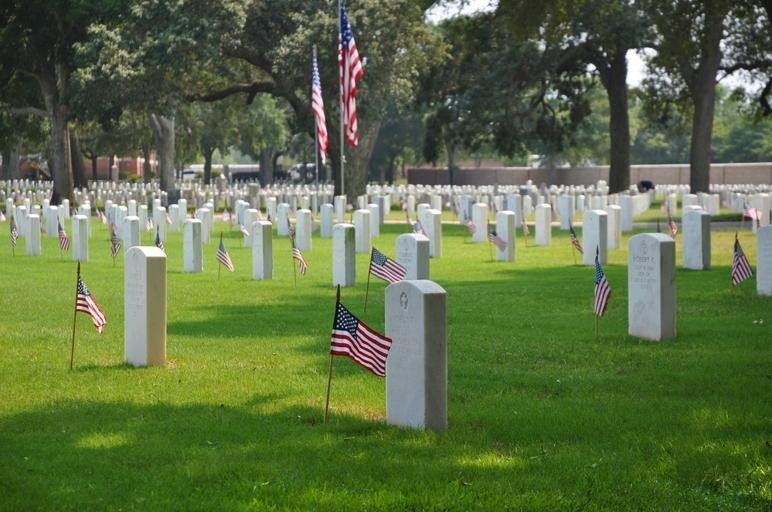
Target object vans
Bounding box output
[287,162,317,184]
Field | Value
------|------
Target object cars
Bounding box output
[176,169,197,180]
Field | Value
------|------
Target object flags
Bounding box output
[464,214,476,232]
[10,215,18,247]
[288,222,295,239]
[74,271,109,336]
[293,247,307,275]
[239,223,249,237]
[741,200,761,227]
[166,214,171,224]
[310,46,332,166]
[95,206,106,224]
[112,225,121,257]
[337,3,366,149]
[147,218,153,232]
[522,216,529,237]
[311,216,317,233]
[731,239,753,284]
[593,247,614,319]
[332,297,391,376]
[668,206,677,238]
[370,248,407,284]
[75,207,78,215]
[57,220,69,251]
[216,238,234,272]
[156,226,164,252]
[569,225,585,255]
[414,219,424,235]
[488,227,506,253]
